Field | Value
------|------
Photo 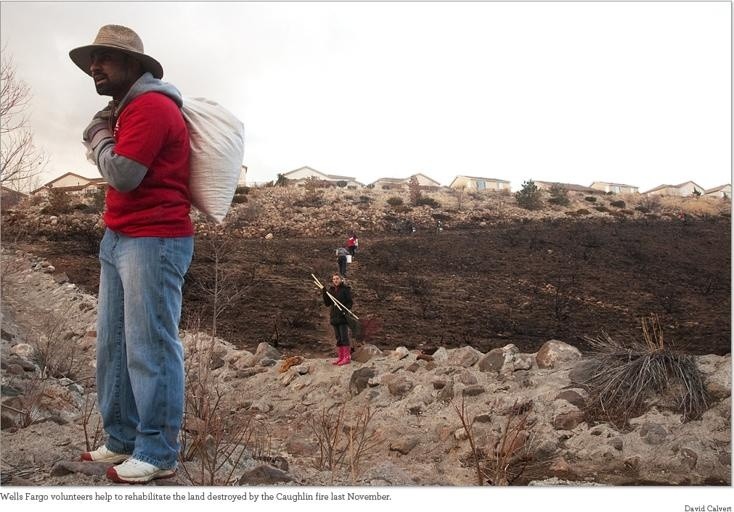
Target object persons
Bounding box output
[348,237,355,255]
[353,234,358,254]
[322,273,353,366]
[336,246,350,276]
[437,220,442,232]
[68,25,195,483]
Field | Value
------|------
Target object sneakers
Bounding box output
[106,456,177,485]
[80,443,132,465]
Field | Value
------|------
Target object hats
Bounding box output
[70,25,163,79]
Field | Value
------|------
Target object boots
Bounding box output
[337,345,352,366]
[332,346,344,365]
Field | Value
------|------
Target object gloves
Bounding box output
[82,107,115,149]
